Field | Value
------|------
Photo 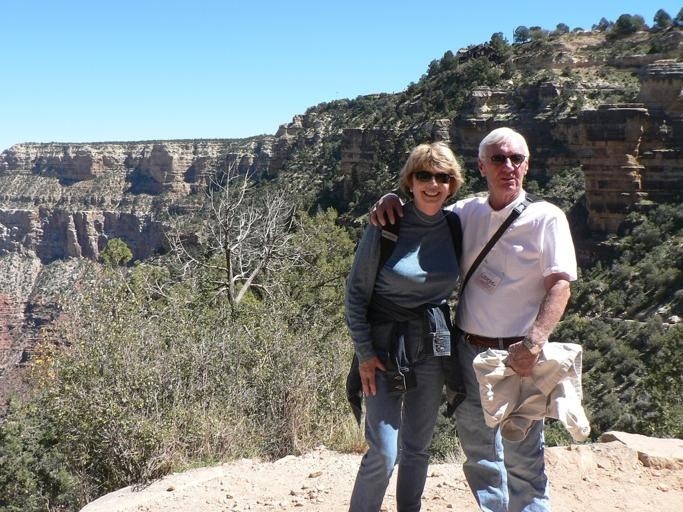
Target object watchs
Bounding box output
[522,338,541,355]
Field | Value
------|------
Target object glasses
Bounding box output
[488,153,526,165]
[411,170,454,184]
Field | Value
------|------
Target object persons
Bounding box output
[367,127,577,512]
[344,141,463,512]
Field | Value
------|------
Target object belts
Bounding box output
[462,332,524,351]
